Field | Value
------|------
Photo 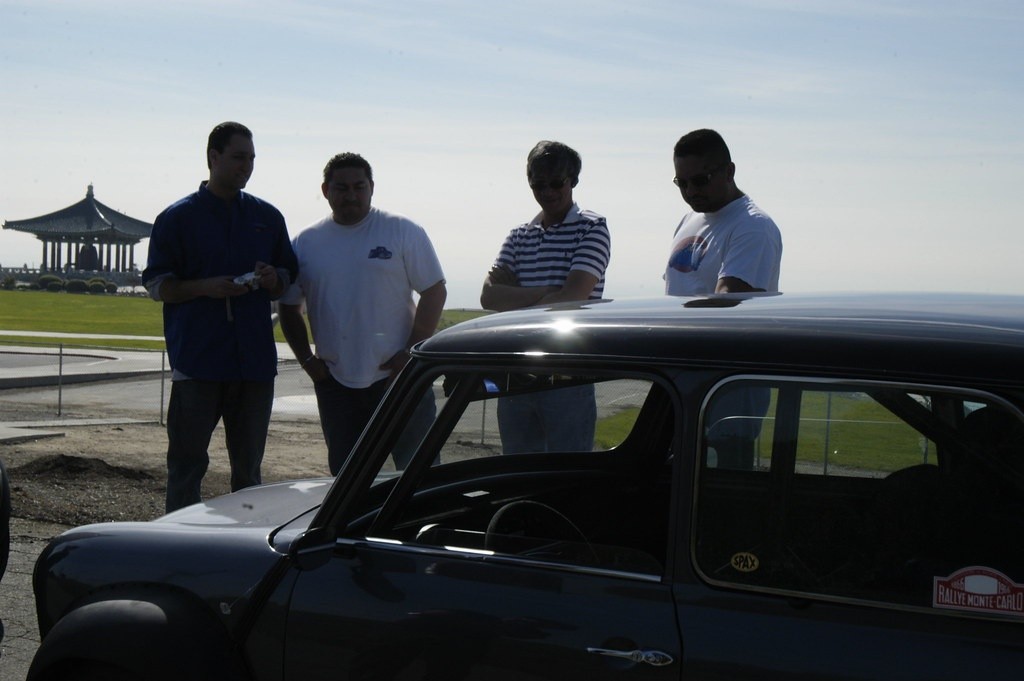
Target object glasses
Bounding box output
[674,162,729,188]
[530,174,570,191]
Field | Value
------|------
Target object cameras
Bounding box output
[234,272,259,290]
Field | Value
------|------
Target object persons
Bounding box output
[480,141,611,455]
[662,128,783,474]
[141,121,301,515]
[280,151,447,478]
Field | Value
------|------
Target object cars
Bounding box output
[28,287,1024,681]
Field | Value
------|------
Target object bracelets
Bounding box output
[300,354,315,368]
[403,347,412,357]
